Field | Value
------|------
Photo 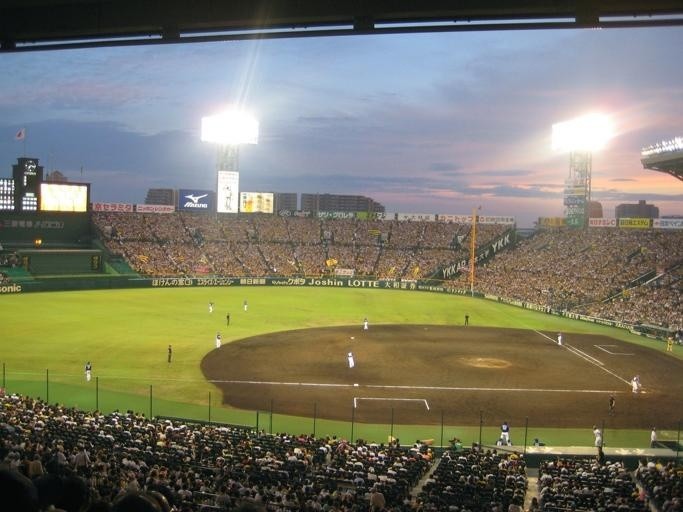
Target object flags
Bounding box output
[13,126,26,140]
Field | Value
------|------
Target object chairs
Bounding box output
[0,392,683,512]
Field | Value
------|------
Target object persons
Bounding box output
[84,360,92,382]
[0,389,682,512]
[167,344,172,363]
[92,208,517,282]
[0,250,24,283]
[243,298,247,311]
[208,301,214,313]
[216,331,221,348]
[443,224,681,353]
[225,311,230,327]
[363,317,369,332]
[629,373,641,395]
